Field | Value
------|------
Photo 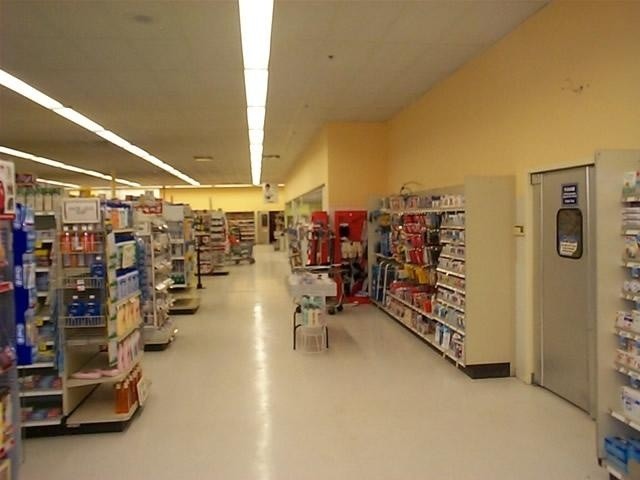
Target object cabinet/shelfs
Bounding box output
[0,159,258,479]
[273,175,516,379]
[594,149,640,479]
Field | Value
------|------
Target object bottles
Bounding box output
[114,361,142,414]
[60,225,97,254]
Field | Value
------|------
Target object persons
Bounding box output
[264,184,275,201]
